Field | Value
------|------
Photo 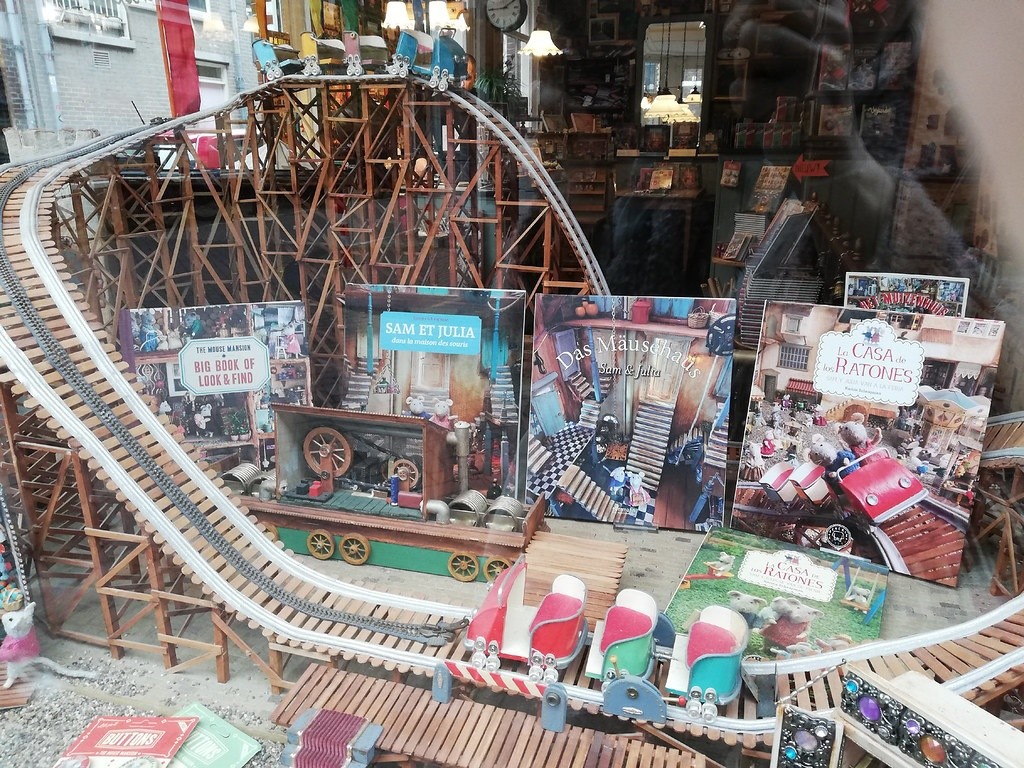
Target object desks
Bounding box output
[616,184,707,288]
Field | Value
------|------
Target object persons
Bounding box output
[719,0,1004,320]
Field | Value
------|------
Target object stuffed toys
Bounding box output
[404,395,453,428]
[847,586,871,615]
[140,311,301,359]
[741,394,888,496]
[707,551,736,577]
[608,467,651,506]
[898,408,932,477]
[201,404,215,437]
[0,602,96,690]
[726,590,825,653]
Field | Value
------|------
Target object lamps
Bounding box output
[644,27,703,112]
[644,18,698,124]
[516,0,565,56]
[383,0,470,33]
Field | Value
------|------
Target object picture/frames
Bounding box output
[587,16,619,45]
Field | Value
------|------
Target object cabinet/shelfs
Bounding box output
[505,0,642,281]
[705,0,925,298]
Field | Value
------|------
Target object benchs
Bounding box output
[758,461,833,515]
[533,576,749,702]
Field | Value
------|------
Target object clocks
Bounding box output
[487,0,528,32]
